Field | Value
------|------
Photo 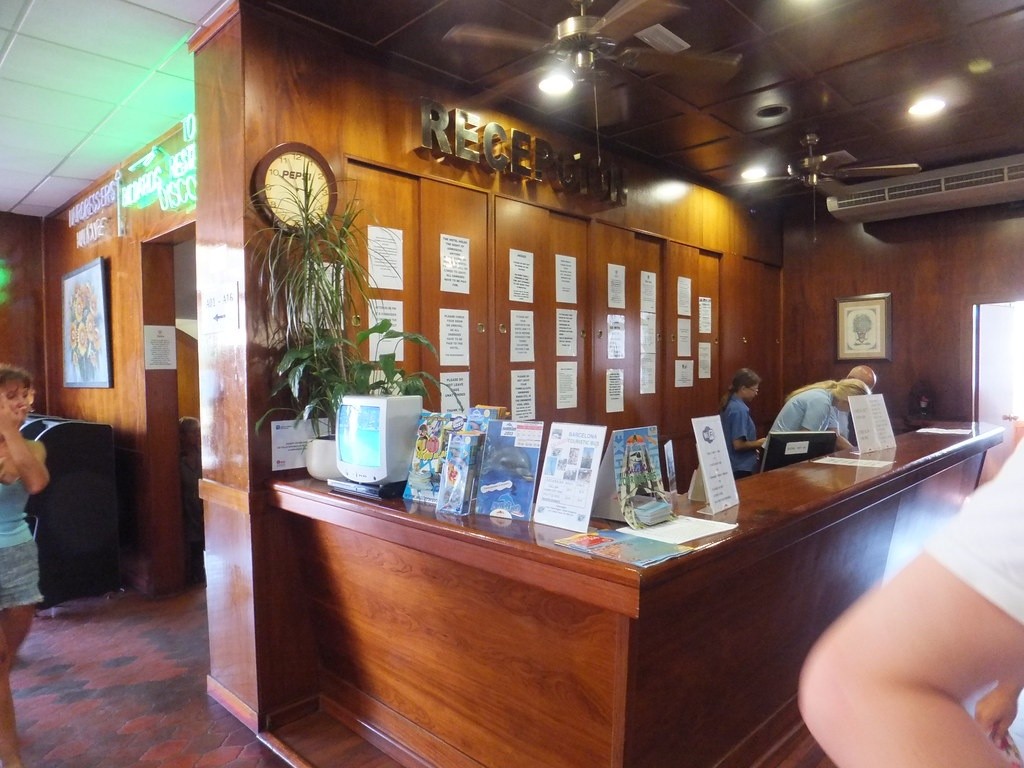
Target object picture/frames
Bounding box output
[836,289,893,363]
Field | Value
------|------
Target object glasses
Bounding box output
[745,384,758,395]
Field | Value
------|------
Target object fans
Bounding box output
[441,0,737,99]
[721,131,921,200]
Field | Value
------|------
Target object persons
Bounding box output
[0,365,50,768]
[718,368,767,479]
[759,378,872,471]
[798,430,1024,768]
[179,416,204,577]
[827,365,877,452]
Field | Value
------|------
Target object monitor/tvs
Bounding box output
[334,394,423,497]
[760,431,838,473]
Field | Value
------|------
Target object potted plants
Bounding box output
[249,157,462,482]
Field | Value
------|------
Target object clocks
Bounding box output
[252,141,338,236]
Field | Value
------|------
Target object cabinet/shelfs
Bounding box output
[19,415,114,595]
